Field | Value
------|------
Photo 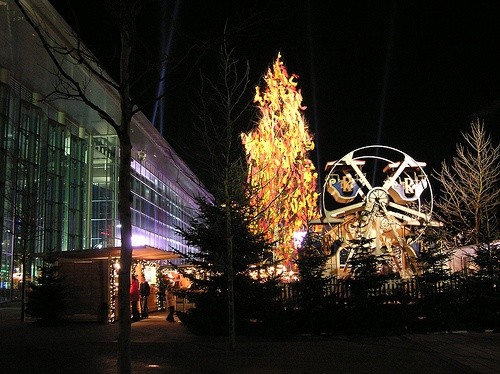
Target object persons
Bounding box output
[130,276,141,322]
[139,274,151,319]
